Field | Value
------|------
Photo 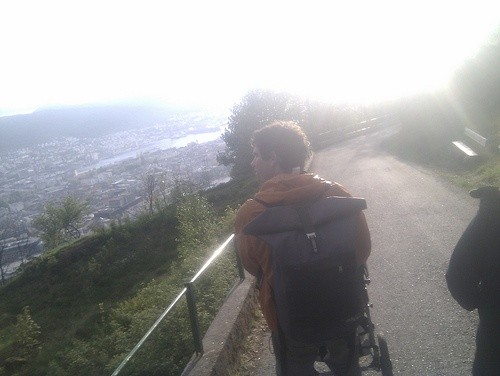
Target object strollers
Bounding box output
[317,265,393,376]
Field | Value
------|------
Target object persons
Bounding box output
[442,186,499,376]
[233,120,372,376]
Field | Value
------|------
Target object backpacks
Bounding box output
[241,174,367,342]
